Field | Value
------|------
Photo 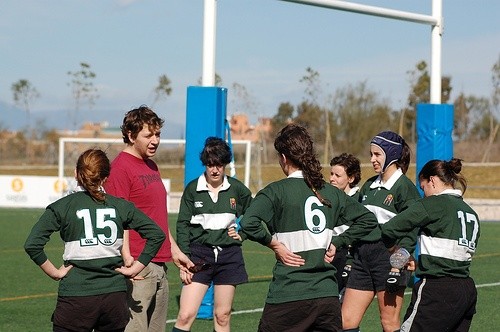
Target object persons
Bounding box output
[102,108,195,332]
[239,124,377,332]
[24,150,165,332]
[341,130,422,332]
[173,138,253,331]
[381,159,480,332]
[330,153,360,196]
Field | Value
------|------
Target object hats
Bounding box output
[369,130,403,172]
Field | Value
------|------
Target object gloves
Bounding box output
[385,271,401,293]
[342,265,352,284]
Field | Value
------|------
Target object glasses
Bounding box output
[188,263,212,273]
